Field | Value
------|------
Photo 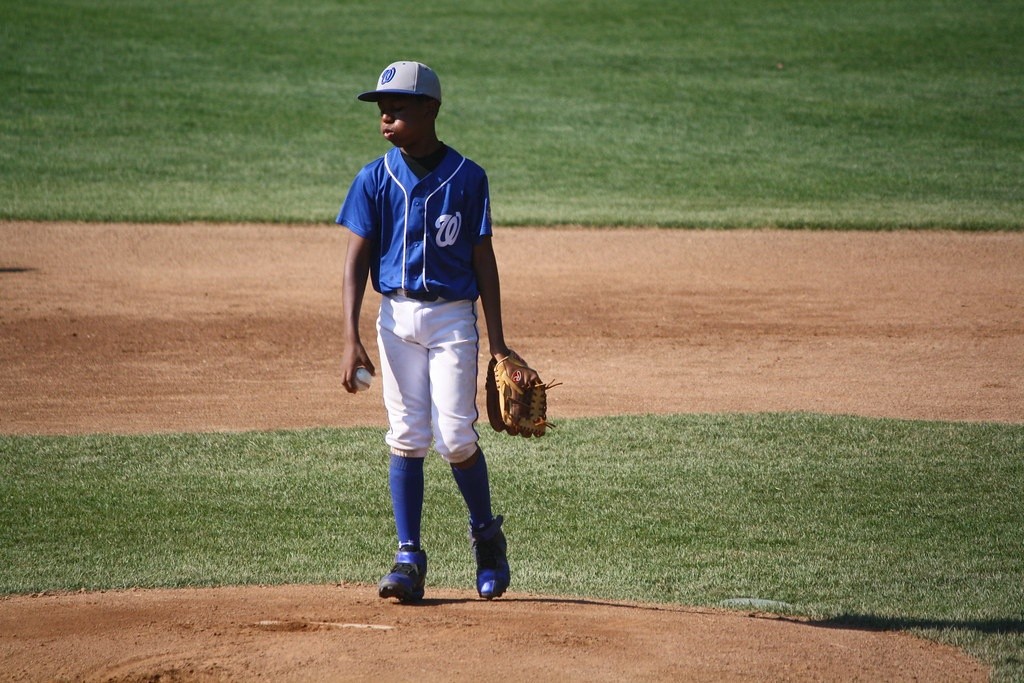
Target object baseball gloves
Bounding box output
[484,349,548,438]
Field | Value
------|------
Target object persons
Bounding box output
[334,62,512,606]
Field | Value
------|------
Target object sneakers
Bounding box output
[467,514,511,599]
[379,540,427,602]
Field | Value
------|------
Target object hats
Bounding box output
[358,61,442,102]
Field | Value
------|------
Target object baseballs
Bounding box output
[353,366,372,393]
